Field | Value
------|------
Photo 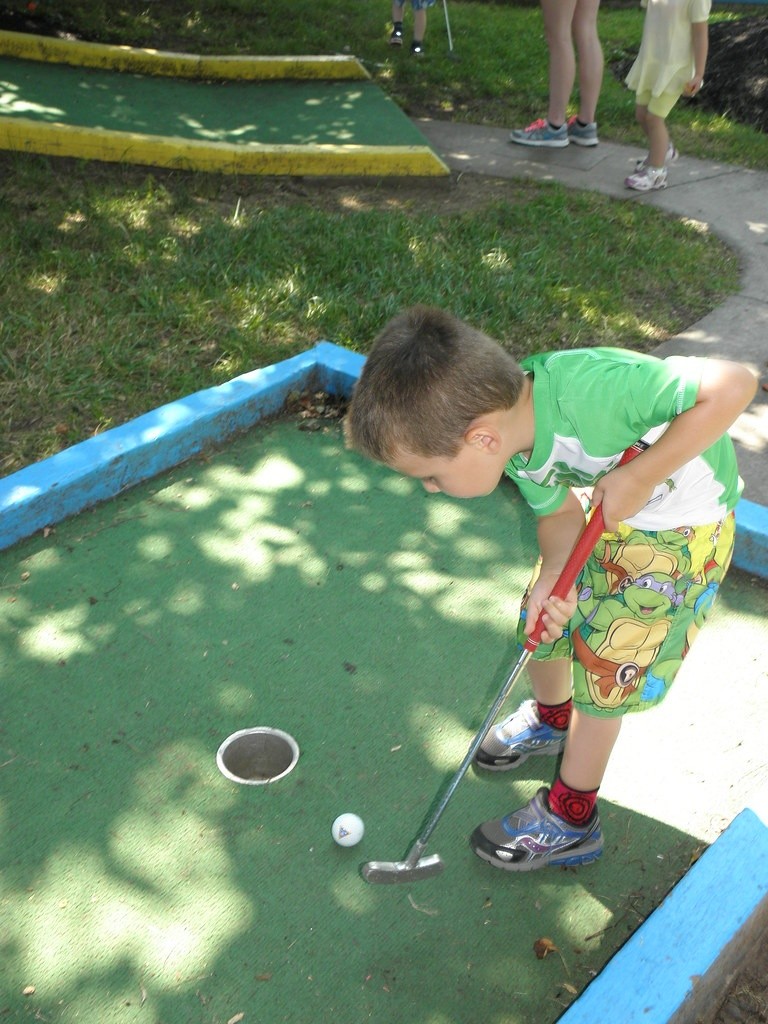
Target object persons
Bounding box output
[510,0,605,148]
[625,0,709,192]
[389,0,427,56]
[350,304,757,874]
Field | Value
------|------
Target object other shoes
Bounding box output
[409,46,423,58]
[389,30,403,45]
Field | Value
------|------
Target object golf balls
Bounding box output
[332,811,366,847]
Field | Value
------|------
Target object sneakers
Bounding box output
[635,143,679,172]
[470,699,568,772]
[470,787,604,871]
[509,119,570,148]
[567,115,599,147]
[625,165,668,191]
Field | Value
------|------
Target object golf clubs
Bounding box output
[359,437,650,885]
